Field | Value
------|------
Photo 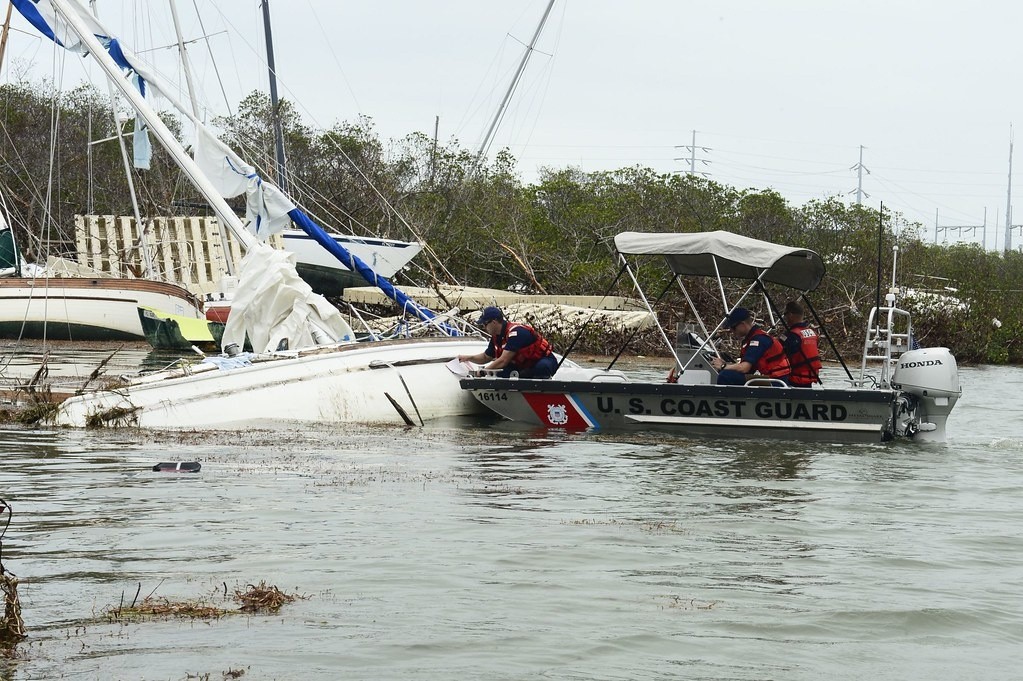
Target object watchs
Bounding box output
[720,362,727,370]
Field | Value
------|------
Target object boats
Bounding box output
[0,0,977,476]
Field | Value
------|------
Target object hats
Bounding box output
[476,306,501,324]
[722,307,750,327]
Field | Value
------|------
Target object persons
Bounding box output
[455,306,558,379]
[713,308,792,387]
[776,301,823,387]
